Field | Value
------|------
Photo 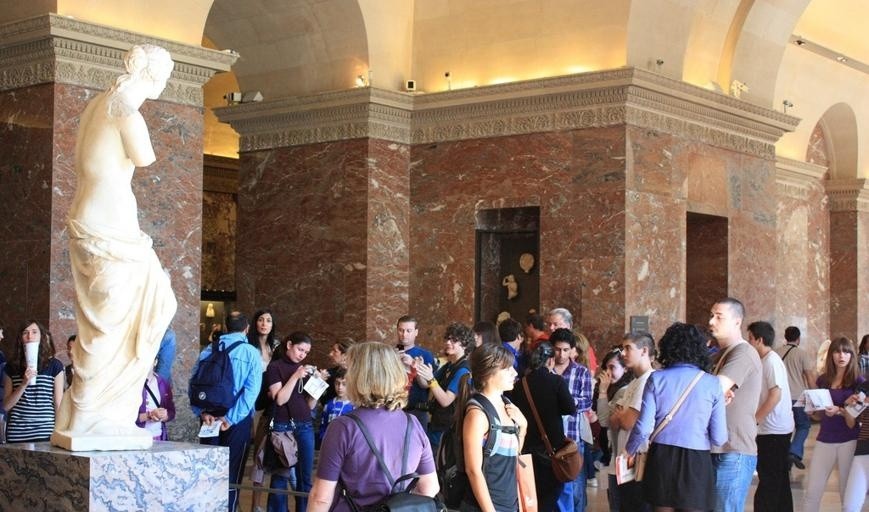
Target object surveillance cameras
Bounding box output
[222,89,229,104]
[444,71,453,90]
[784,99,793,107]
[657,59,664,66]
[736,81,751,94]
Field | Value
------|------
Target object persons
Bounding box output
[1,296,869,512]
[47,45,177,454]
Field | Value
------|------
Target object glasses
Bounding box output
[612,344,623,352]
[443,336,459,344]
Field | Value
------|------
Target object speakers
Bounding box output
[230,92,242,101]
[406,79,415,90]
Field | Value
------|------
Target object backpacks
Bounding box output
[436,393,526,512]
[188,330,248,417]
[337,412,447,512]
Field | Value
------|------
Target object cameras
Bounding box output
[304,364,316,376]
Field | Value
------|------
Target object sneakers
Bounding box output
[587,460,603,487]
[787,454,805,470]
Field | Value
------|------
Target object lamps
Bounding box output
[354,74,367,90]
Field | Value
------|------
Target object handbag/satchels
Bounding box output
[549,438,584,483]
[515,453,539,512]
[634,452,648,482]
[264,393,305,468]
